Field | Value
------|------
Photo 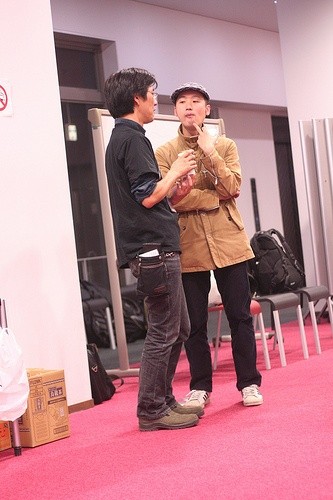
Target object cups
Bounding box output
[178,150,195,175]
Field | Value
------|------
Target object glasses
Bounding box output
[147,91,158,98]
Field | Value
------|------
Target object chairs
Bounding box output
[206,285,333,370]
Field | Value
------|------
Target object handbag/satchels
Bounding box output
[136,243,170,296]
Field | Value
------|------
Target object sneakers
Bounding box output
[183,390,210,407]
[139,408,199,431]
[172,403,204,417]
[241,384,263,406]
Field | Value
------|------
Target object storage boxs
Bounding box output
[0,368,72,452]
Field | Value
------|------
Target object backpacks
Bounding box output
[87,343,124,404]
[80,279,148,348]
[250,229,306,297]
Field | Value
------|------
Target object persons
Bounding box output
[154,81,263,407]
[103,67,206,432]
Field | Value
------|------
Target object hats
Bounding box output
[171,82,210,104]
